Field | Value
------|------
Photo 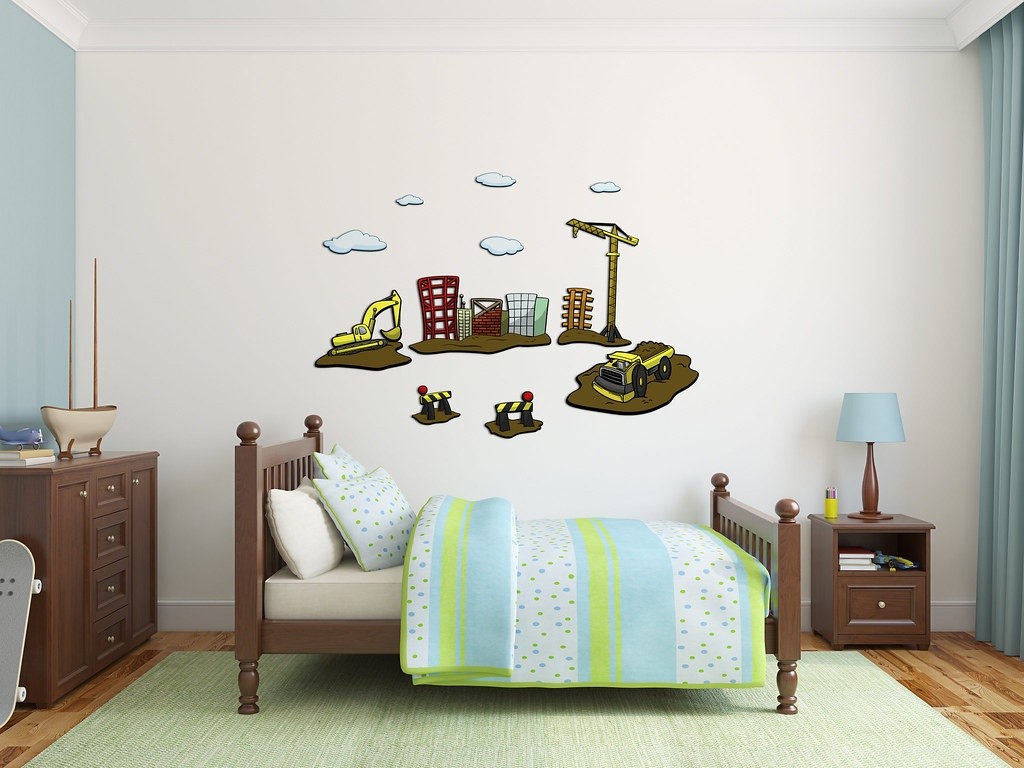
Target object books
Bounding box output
[0,449,54,459]
[838,546,878,571]
[0,456,55,466]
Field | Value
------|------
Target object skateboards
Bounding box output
[0,539,43,729]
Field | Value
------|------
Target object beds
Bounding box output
[234,415,802,715]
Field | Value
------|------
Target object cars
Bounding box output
[872,551,920,570]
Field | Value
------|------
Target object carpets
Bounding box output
[19,652,1017,768]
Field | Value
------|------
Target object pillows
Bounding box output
[312,443,369,480]
[313,467,418,573]
[265,476,344,580]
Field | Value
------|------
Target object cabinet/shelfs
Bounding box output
[0,451,160,710]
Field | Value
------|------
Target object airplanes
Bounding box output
[0,426,48,450]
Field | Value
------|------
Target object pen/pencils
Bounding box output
[824,486,838,498]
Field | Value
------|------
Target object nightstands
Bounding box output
[807,514,936,650]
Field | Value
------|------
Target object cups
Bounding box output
[824,499,839,518]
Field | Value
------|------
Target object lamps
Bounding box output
[835,393,905,520]
